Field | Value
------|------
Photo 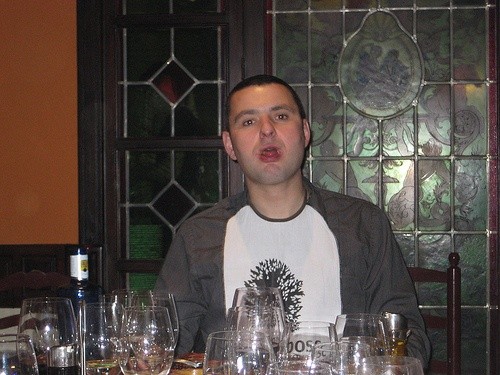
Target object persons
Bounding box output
[128,74,432,375]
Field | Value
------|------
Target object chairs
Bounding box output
[404,251,461,375]
[0,270,108,341]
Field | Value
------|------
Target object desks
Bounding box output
[0,349,384,375]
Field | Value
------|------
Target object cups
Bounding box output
[0,286,179,375]
[203,285,423,375]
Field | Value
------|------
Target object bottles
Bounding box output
[59,248,98,359]
